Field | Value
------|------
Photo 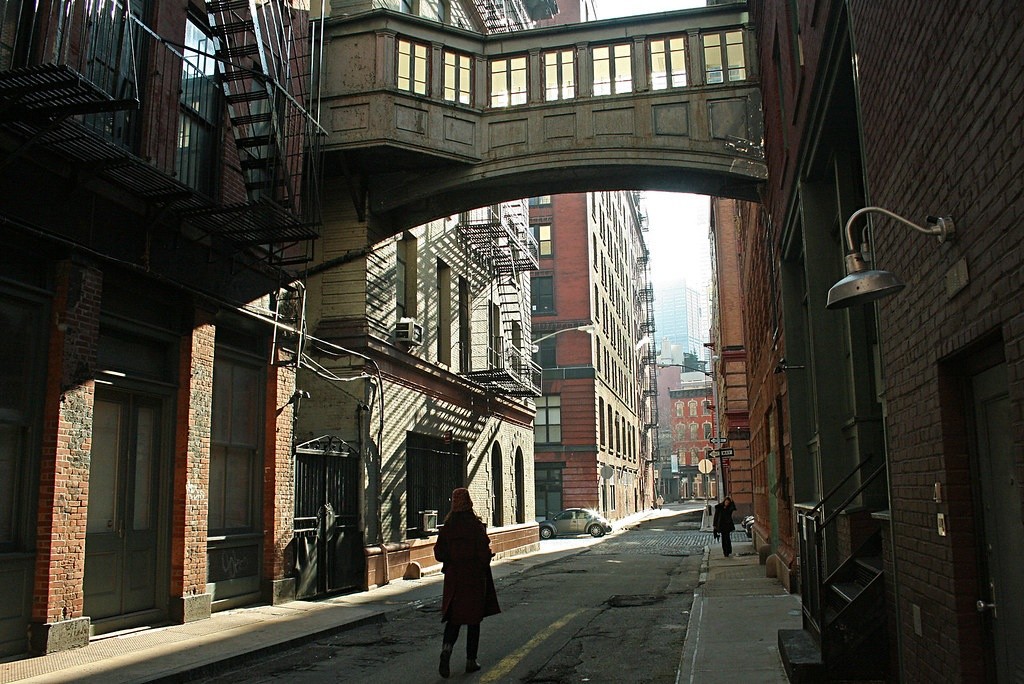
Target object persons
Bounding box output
[655,495,664,510]
[433,487,502,678]
[713,497,738,558]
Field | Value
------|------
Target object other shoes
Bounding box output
[729,548,732,554]
[724,552,729,557]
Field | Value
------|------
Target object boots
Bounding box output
[438,643,452,679]
[465,659,481,673]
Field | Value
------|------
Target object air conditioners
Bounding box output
[395,322,423,346]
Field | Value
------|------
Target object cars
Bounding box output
[537,508,613,539]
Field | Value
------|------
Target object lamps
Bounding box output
[824,206,956,312]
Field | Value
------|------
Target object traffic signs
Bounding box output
[706,437,734,459]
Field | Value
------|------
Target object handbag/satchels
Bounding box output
[712,528,720,543]
